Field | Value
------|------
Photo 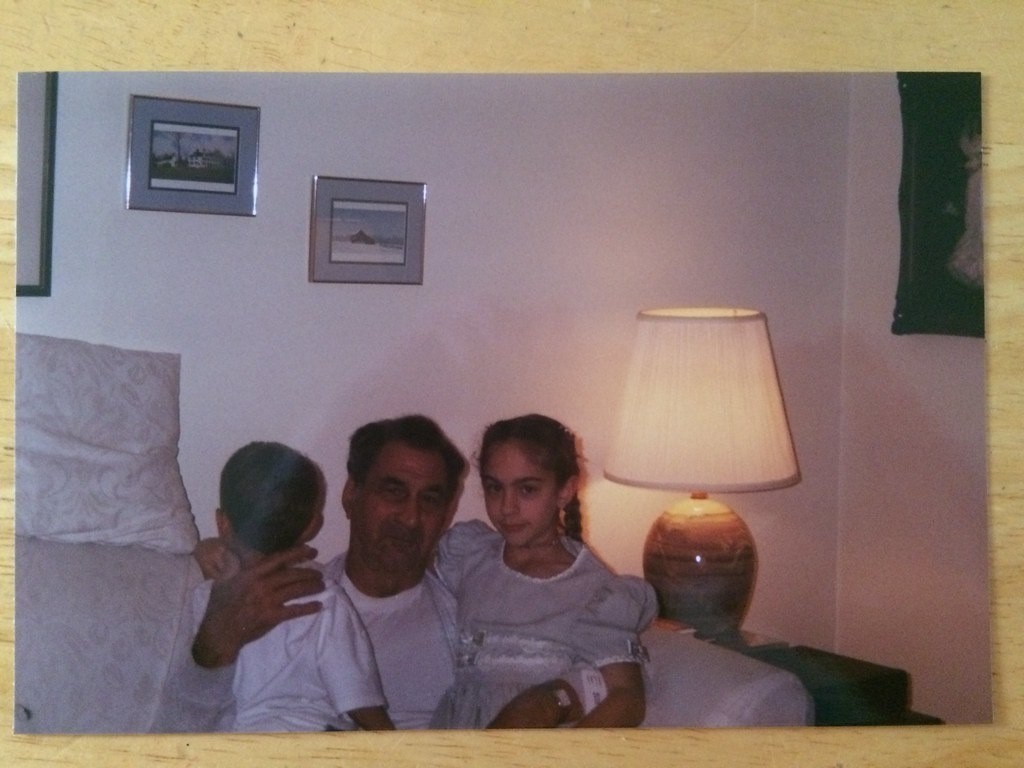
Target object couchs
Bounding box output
[16,545,815,728]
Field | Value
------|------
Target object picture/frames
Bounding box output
[125,94,262,219]
[308,175,428,285]
[16,72,59,298]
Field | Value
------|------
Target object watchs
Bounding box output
[544,682,571,722]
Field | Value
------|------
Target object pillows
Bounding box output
[17,332,200,555]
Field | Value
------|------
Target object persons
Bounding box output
[194,439,397,732]
[190,414,606,729]
[324,414,658,729]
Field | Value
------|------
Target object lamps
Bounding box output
[603,308,800,640]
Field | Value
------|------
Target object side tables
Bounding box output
[695,630,788,657]
